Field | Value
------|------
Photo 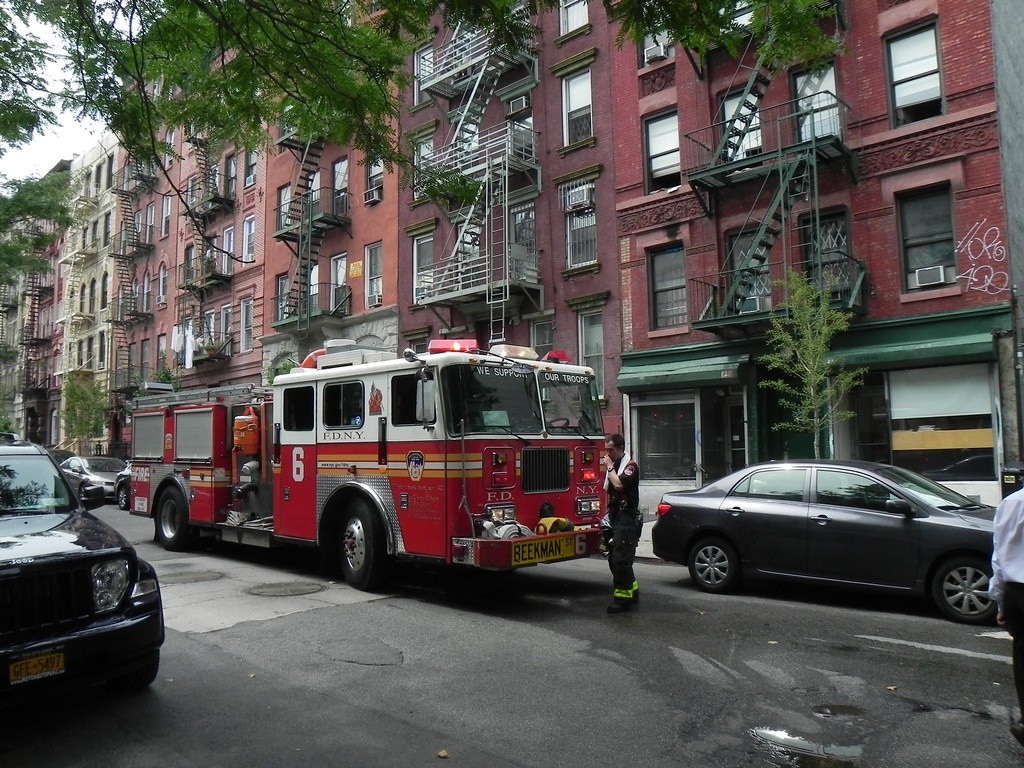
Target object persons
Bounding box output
[601,433,644,614]
[988,485,1024,744]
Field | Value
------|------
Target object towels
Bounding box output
[602,451,631,493]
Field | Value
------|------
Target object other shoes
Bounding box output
[1010,720,1024,747]
[631,590,639,603]
[607,597,632,613]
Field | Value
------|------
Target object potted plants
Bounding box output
[202,344,223,355]
[823,269,852,292]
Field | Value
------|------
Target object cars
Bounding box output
[112,460,133,511]
[651,460,1003,627]
[58,455,129,503]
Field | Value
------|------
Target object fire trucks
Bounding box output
[131,340,615,591]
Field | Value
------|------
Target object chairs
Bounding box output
[827,477,850,507]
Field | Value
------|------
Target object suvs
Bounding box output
[0,432,167,704]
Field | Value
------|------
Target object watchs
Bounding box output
[607,465,615,472]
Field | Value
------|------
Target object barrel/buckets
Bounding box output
[234,416,258,455]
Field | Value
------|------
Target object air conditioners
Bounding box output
[570,188,592,207]
[156,295,167,304]
[368,294,383,307]
[246,175,256,185]
[915,265,946,287]
[644,43,669,64]
[98,362,105,370]
[510,97,529,111]
[245,254,253,264]
[364,189,382,204]
